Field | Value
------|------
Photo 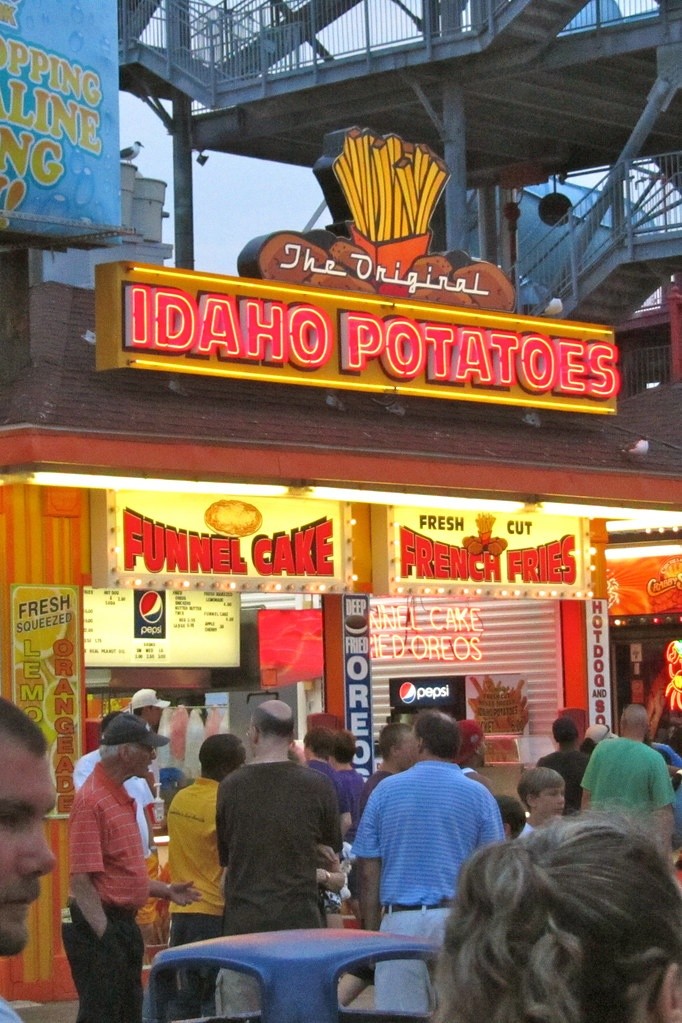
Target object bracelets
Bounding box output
[324,870,331,882]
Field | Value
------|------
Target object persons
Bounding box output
[69,689,248,944]
[0,697,57,1023]
[430,811,682,1023]
[513,716,682,871]
[578,704,674,868]
[214,700,343,1016]
[60,714,202,1023]
[350,708,505,1014]
[217,697,527,1005]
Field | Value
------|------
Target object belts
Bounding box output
[385,900,455,914]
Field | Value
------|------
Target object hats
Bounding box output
[132,689,171,708]
[101,713,171,748]
[454,719,483,765]
[585,724,619,745]
